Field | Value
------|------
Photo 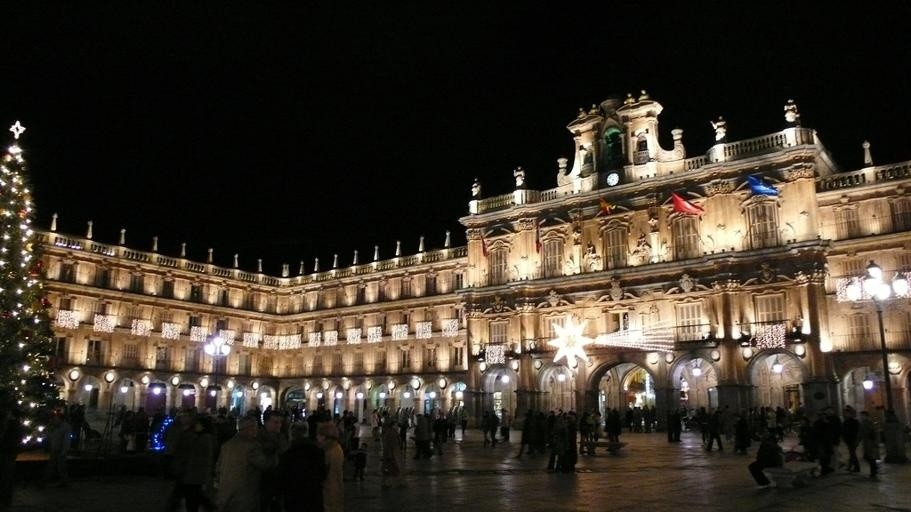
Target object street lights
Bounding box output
[203,336,232,413]
[845,256,910,465]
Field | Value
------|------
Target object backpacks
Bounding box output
[191,435,222,471]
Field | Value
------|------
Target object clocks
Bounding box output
[605,171,622,186]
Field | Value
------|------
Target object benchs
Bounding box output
[763,460,820,489]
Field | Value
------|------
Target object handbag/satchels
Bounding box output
[381,458,400,477]
[861,437,882,461]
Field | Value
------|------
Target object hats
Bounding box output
[800,406,855,423]
[319,424,340,440]
[236,415,263,430]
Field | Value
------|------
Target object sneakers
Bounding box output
[814,463,883,483]
[753,482,769,490]
[379,483,409,490]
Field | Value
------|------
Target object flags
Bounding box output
[749,176,780,197]
[671,194,703,216]
